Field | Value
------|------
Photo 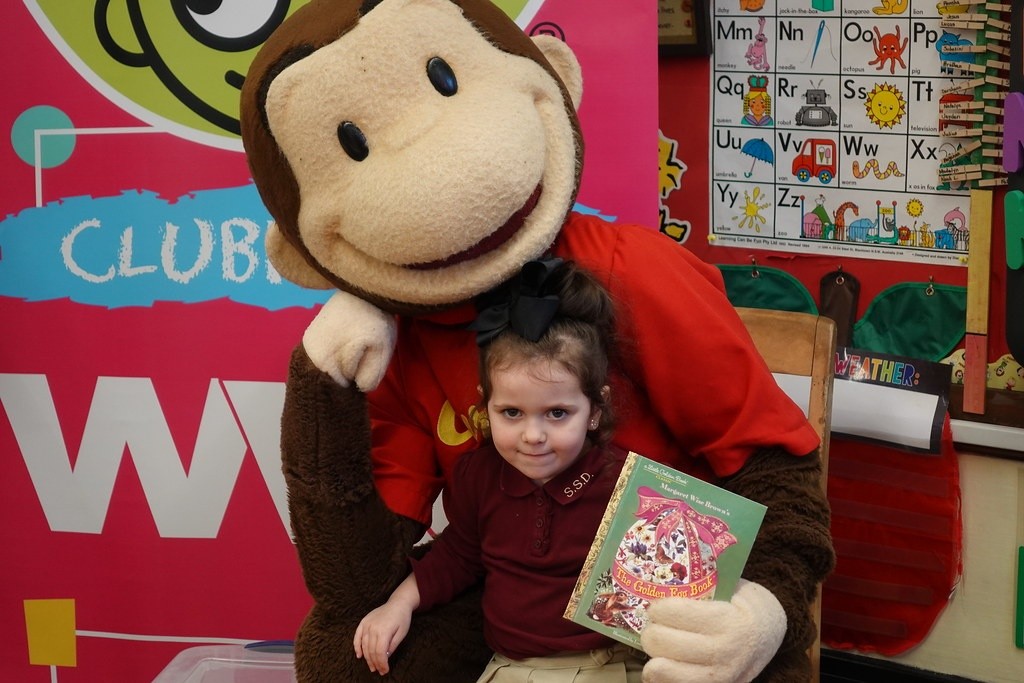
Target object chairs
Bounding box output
[732,305,838,683]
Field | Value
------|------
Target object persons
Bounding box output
[352,257,643,682]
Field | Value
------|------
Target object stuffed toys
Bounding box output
[238,1,835,683]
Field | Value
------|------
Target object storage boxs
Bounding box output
[151,639,297,683]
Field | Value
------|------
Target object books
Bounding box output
[560,449,769,660]
